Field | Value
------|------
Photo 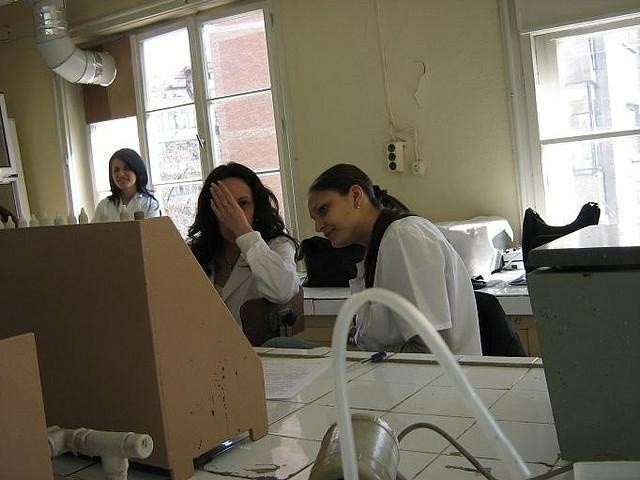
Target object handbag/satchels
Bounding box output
[301,235,366,287]
[521,201,600,281]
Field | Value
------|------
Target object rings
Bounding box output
[222,200,228,206]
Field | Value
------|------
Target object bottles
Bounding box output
[0,204,131,231]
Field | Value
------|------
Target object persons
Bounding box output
[89,148,161,219]
[308,161,483,356]
[182,159,305,334]
